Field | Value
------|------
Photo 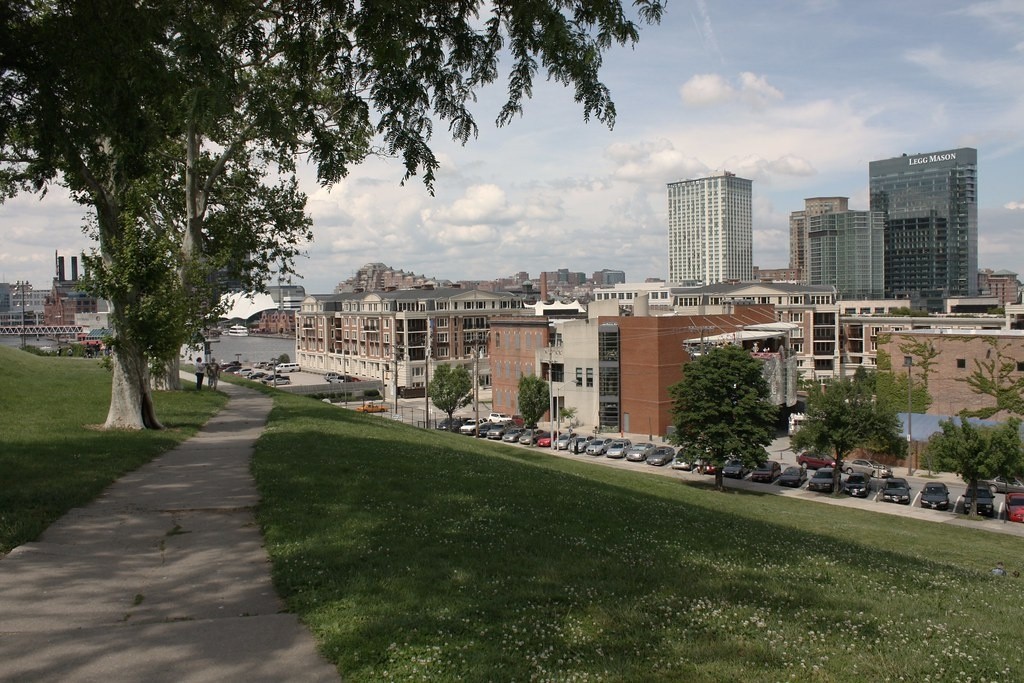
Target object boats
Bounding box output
[222,330,229,336]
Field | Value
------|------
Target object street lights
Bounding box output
[12,280,33,349]
[556,379,578,450]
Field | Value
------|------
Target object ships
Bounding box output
[229,324,248,337]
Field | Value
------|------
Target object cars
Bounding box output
[1004,493,1024,522]
[537,431,561,447]
[626,443,656,461]
[920,481,950,511]
[809,467,841,492]
[646,446,674,466]
[777,466,807,487]
[585,438,612,454]
[606,439,632,458]
[568,436,595,452]
[881,477,911,504]
[841,459,894,478]
[554,432,578,450]
[519,429,544,444]
[844,472,871,497]
[355,404,388,413]
[438,413,525,443]
[987,474,1024,493]
[798,451,840,469]
[671,444,751,479]
[752,461,781,483]
[324,372,360,384]
[221,361,299,385]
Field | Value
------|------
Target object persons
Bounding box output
[194,357,206,390]
[86,340,100,358]
[56,346,63,357]
[207,357,221,390]
[67,347,74,356]
[105,341,112,354]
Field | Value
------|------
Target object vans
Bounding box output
[962,480,996,517]
